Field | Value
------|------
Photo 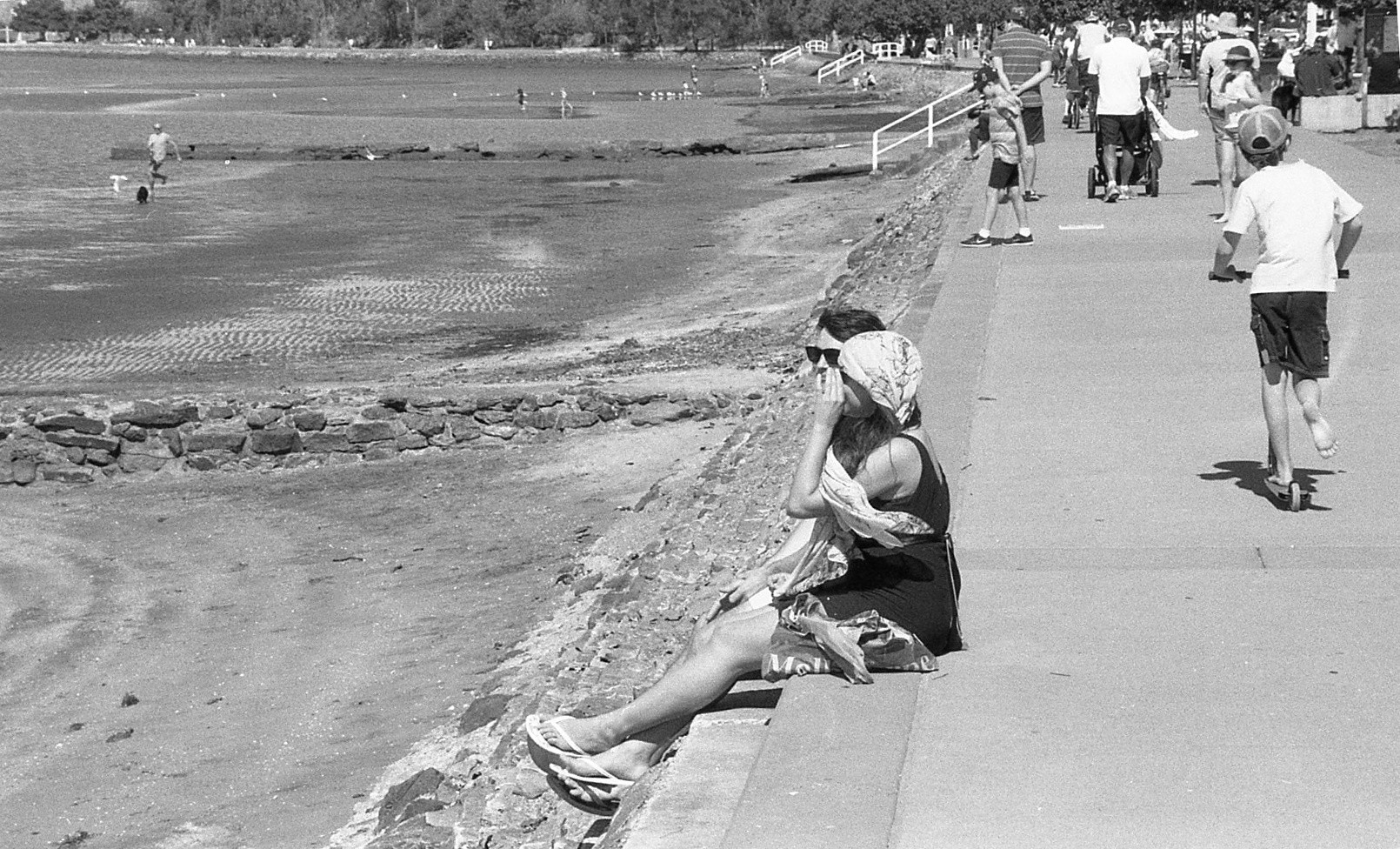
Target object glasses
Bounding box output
[805,346,842,368]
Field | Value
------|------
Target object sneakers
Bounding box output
[959,233,992,248]
[1001,234,1033,247]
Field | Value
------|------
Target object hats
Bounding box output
[1113,18,1132,32]
[1209,12,1245,35]
[1236,103,1292,154]
[967,67,998,93]
[1221,45,1253,61]
[1005,7,1025,21]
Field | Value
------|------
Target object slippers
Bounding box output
[546,774,619,817]
[526,713,590,759]
[549,757,635,786]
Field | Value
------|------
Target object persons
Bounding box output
[691,65,698,84]
[559,88,573,110]
[758,75,769,98]
[1212,104,1365,487]
[842,43,849,55]
[147,123,182,191]
[526,303,962,818]
[853,71,876,92]
[515,87,528,110]
[907,7,1400,223]
[960,66,1034,247]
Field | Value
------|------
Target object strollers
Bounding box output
[1067,64,1098,133]
[1087,89,1160,197]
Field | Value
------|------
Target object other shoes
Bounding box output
[1118,188,1139,200]
[1022,191,1039,201]
[1103,186,1120,203]
[1164,89,1171,98]
